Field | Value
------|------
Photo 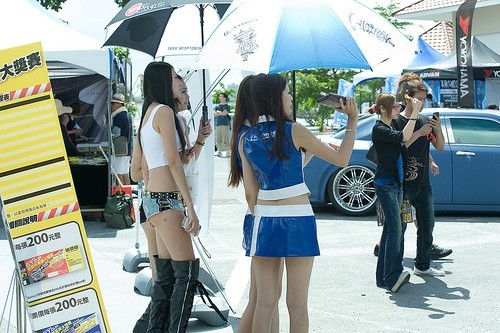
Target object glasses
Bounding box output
[390,104,402,110]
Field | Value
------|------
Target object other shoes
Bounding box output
[215,152,221,156]
[226,152,230,158]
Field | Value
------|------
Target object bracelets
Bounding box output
[345,129,357,133]
[409,117,418,120]
[196,141,205,146]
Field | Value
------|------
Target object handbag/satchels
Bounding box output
[112,136,128,156]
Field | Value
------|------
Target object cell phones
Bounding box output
[433,112,439,120]
[182,216,201,236]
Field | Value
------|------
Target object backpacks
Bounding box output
[104,191,133,228]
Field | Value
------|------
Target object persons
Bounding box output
[213,92,231,159]
[102,92,133,186]
[367,92,411,293]
[393,73,455,276]
[53,98,88,158]
[191,0,420,122]
[425,94,499,111]
[372,100,453,261]
[130,60,200,333]
[132,72,213,333]
[227,74,326,332]
[239,71,359,333]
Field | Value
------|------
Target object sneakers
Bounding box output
[414,265,445,276]
[374,245,380,256]
[431,246,453,259]
[391,272,410,293]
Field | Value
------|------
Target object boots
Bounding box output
[145,254,175,333]
[168,258,200,333]
[133,302,151,333]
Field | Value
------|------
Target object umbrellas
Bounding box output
[100,0,237,135]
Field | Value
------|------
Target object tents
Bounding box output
[354,36,448,112]
[401,34,500,107]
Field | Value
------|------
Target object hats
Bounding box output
[111,94,125,104]
[54,99,73,116]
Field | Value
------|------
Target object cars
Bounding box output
[301,107,500,217]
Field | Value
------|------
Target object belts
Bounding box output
[143,190,183,201]
